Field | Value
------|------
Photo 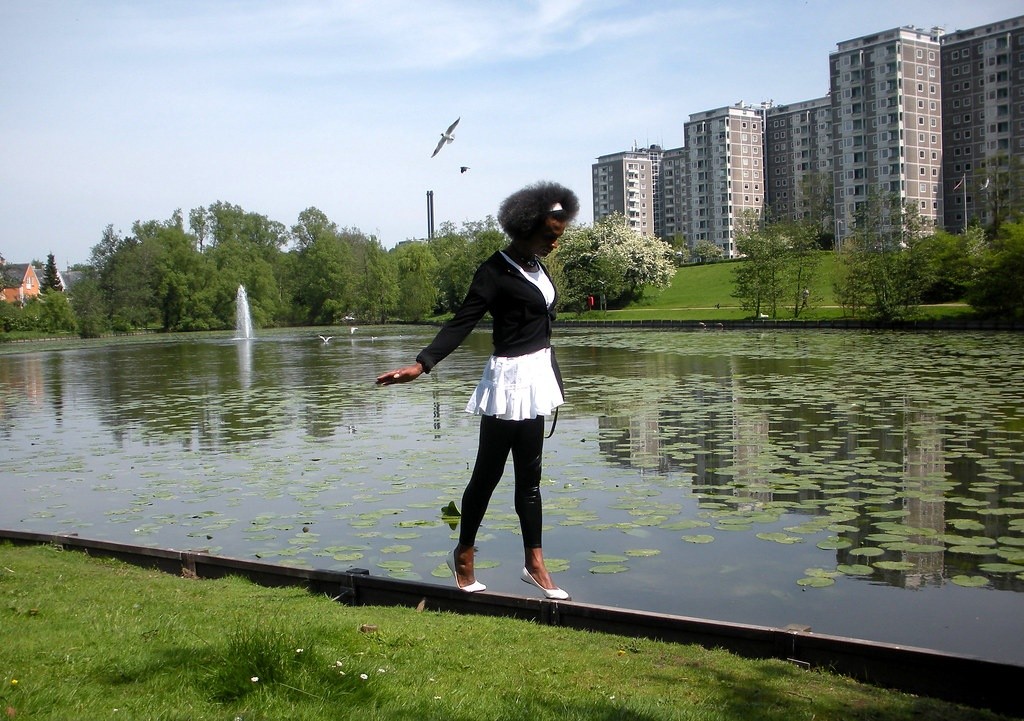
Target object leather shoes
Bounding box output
[446,550,487,593]
[520,567,569,600]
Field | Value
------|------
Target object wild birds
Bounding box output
[431,115,461,159]
[317,334,333,344]
[344,315,359,336]
[459,164,471,176]
[370,336,378,342]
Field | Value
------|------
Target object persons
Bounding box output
[375,180,580,600]
[802,286,810,307]
